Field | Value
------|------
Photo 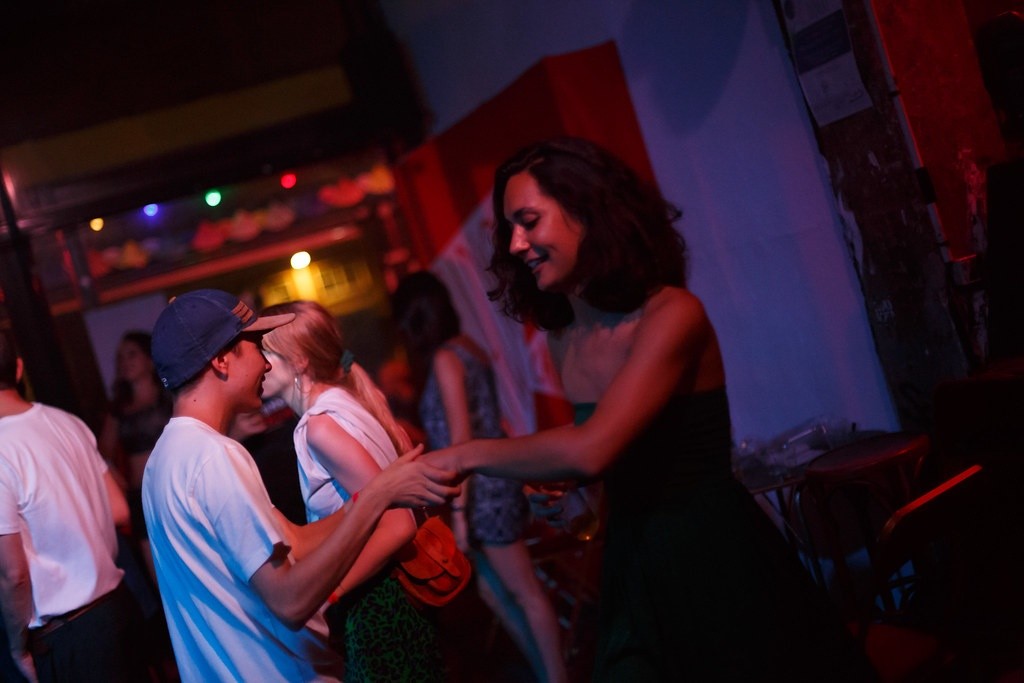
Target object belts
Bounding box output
[38,592,110,639]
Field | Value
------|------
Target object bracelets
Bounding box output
[352,490,359,503]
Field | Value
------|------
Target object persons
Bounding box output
[390,137,881,683]
[0,327,179,683]
[141,270,568,683]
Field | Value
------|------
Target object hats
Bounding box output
[150,287,294,391]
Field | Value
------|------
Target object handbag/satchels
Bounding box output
[387,515,473,620]
